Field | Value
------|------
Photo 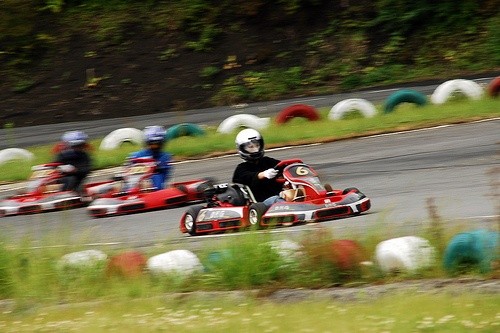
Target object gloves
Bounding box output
[264,168,279,179]
[56,165,76,172]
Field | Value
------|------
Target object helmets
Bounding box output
[235,129,264,161]
[62,131,89,146]
[142,125,167,142]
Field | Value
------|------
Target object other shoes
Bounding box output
[322,183,334,197]
[293,189,304,203]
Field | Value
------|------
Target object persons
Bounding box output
[232,128,289,205]
[52,131,89,192]
[124,126,172,191]
